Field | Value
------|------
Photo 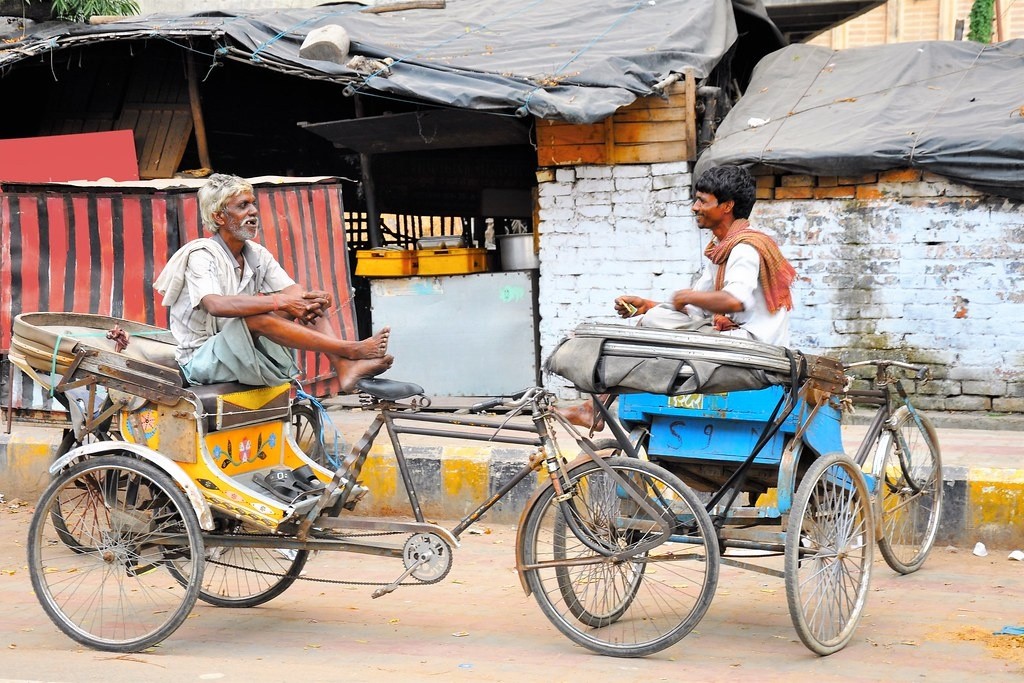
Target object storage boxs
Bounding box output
[354,249,418,277]
[417,247,490,276]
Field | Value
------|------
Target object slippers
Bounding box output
[253,470,307,504]
[270,464,327,496]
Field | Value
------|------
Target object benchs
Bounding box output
[185,381,295,429]
[616,390,844,458]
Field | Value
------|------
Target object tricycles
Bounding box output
[5,311,721,658]
[539,304,944,656]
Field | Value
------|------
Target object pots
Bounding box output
[495,233,539,270]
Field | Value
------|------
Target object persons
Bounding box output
[170,174,396,390]
[546,162,796,434]
